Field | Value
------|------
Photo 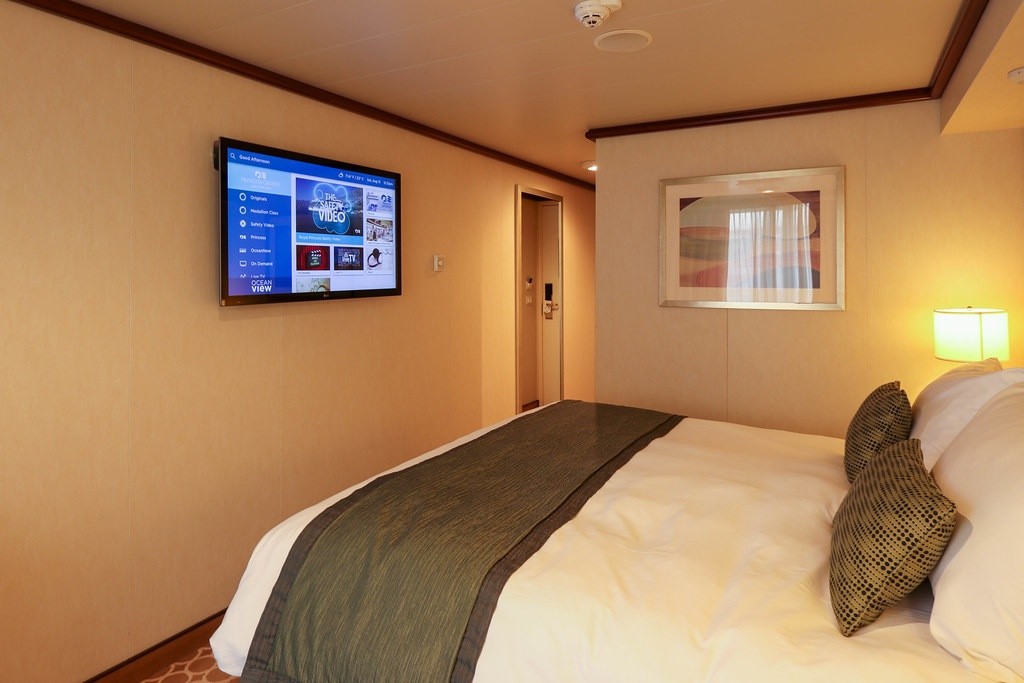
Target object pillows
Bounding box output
[844,380,913,484]
[910,358,1024,473]
[929,380,1024,683]
[828,438,959,638]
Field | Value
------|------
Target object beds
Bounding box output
[209,357,1024,683]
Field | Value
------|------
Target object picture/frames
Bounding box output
[657,165,846,311]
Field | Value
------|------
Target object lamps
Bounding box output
[932,307,1010,362]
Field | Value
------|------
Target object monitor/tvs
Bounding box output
[216,136,402,306]
